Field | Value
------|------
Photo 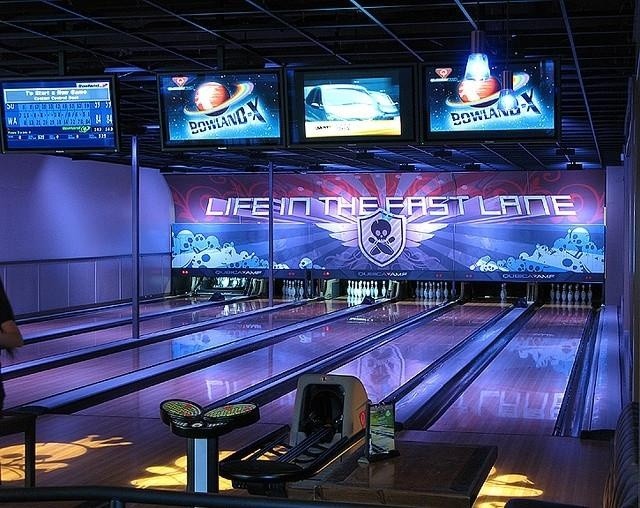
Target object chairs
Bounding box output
[500,400,640,508]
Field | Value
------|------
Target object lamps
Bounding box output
[492,2,522,111]
[463,2,494,81]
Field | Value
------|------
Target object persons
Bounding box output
[0,280,24,412]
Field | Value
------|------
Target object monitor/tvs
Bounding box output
[419,54,562,148]
[0,75,121,154]
[285,63,418,150]
[156,69,287,152]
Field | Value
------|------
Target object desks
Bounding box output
[314,435,500,508]
